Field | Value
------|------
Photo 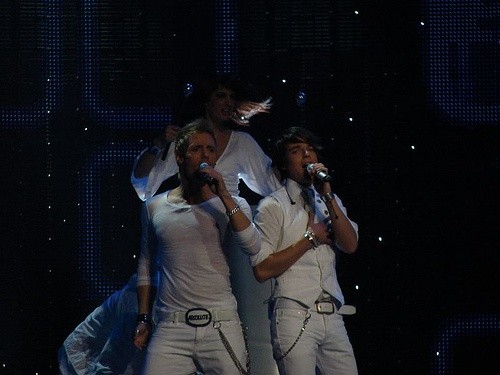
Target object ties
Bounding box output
[301,189,315,216]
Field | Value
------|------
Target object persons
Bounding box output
[58,272,147,375]
[248,126,358,375]
[134,118,261,375]
[131,77,282,375]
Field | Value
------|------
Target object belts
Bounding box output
[275,300,357,315]
[159,308,235,327]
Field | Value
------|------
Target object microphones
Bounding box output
[306,163,331,182]
[200,162,213,181]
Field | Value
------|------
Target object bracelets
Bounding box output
[226,206,239,215]
[136,314,151,324]
[321,192,334,202]
[304,231,319,248]
